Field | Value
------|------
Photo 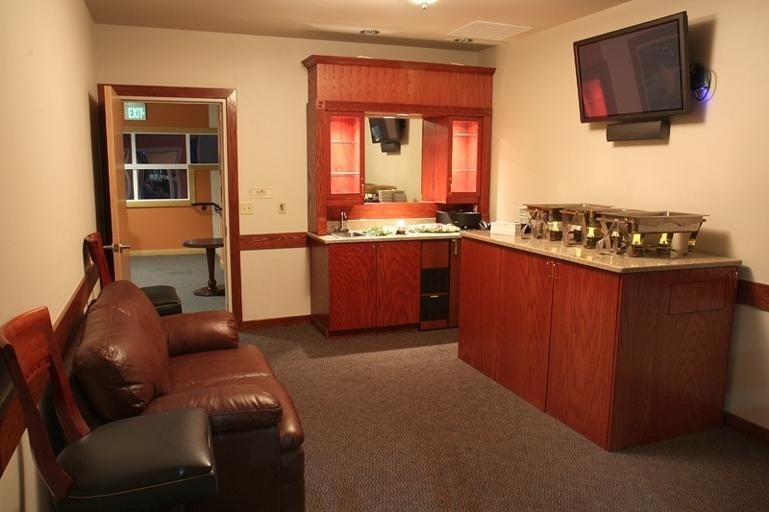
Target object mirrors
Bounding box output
[364,114,424,203]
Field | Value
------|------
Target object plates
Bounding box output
[378,189,405,203]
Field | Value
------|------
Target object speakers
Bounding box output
[379,142,400,153]
[605,122,672,141]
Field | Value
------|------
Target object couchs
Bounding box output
[62,279,305,512]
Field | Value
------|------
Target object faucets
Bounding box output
[340,209,347,232]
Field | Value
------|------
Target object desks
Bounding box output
[182,238,225,296]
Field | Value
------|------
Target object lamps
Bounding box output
[409,0,438,9]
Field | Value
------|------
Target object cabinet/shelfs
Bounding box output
[310,238,461,338]
[324,111,365,206]
[461,237,501,381]
[421,116,483,203]
[500,244,740,451]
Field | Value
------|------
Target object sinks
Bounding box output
[330,230,371,239]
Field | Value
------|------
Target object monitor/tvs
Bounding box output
[572,11,692,125]
[370,116,410,142]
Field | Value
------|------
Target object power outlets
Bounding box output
[240,202,254,214]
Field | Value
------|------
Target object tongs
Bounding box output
[520,208,619,254]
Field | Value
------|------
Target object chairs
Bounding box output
[0,305,217,512]
[85,231,181,317]
